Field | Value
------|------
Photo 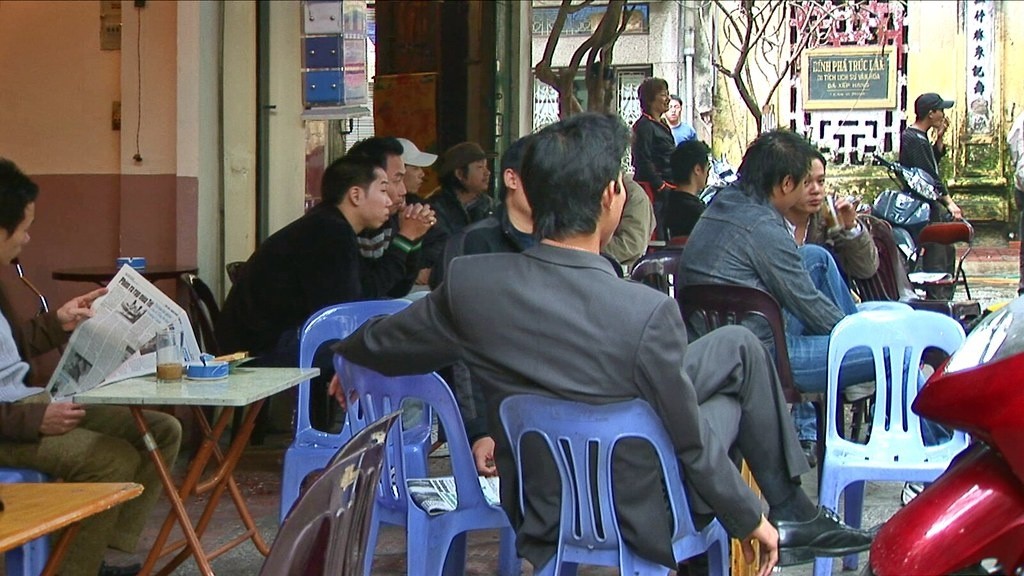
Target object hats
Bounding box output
[445,141,499,171]
[396,138,438,167]
[915,93,954,113]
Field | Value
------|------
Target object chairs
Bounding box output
[282,221,1024,575]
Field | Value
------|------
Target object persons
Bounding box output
[212,78,880,480]
[0,158,183,576]
[677,131,955,510]
[327,111,875,576]
[899,93,963,301]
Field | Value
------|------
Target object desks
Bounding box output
[52,265,199,290]
[0,483,144,576]
[61,365,321,576]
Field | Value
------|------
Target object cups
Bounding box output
[819,194,845,235]
[155,330,182,383]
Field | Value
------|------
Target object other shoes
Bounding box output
[800,440,818,468]
[99,560,141,576]
[426,429,451,458]
[901,480,925,505]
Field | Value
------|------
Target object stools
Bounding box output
[0,466,46,576]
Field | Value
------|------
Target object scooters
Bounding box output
[870,150,949,278]
[863,289,1024,576]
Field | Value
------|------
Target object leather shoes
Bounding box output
[770,504,883,566]
